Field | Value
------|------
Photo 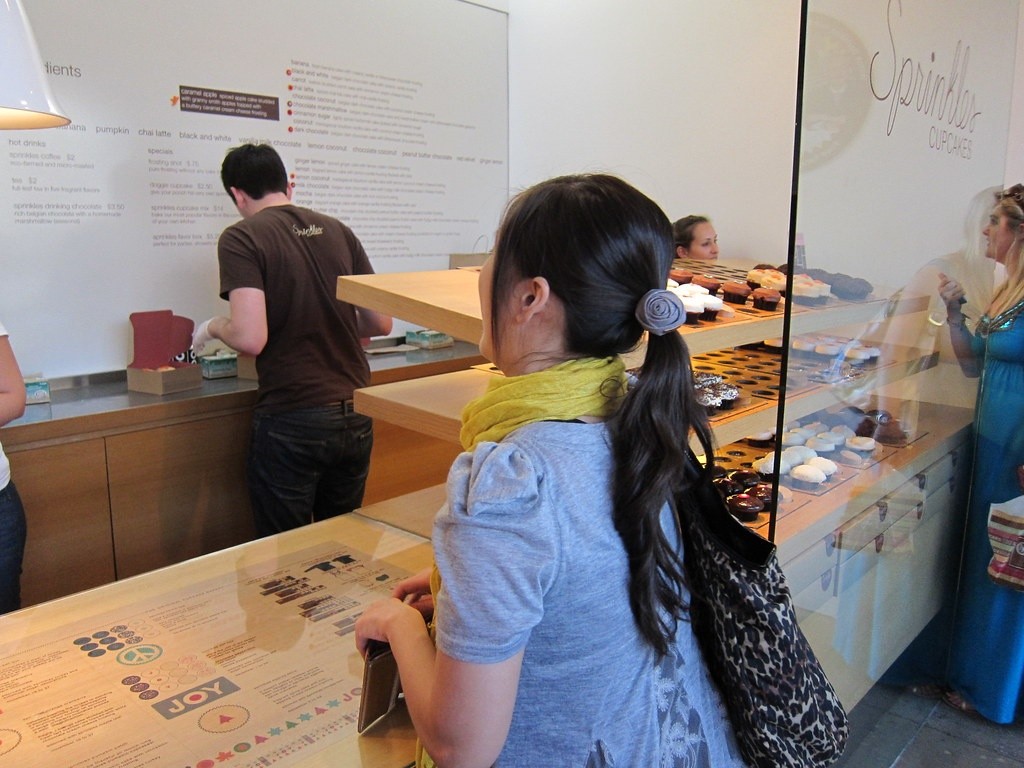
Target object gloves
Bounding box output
[193,317,216,354]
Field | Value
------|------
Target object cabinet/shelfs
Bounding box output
[0,257,937,609]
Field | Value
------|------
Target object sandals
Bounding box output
[941,689,978,713]
[902,679,944,700]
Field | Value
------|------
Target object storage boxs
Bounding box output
[237,351,259,381]
[126,362,204,396]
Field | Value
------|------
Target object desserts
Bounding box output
[740,334,881,369]
[623,367,740,416]
[663,263,874,325]
[156,365,175,372]
[704,405,907,523]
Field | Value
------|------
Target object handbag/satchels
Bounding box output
[824,258,957,414]
[986,491,1024,592]
[831,459,924,551]
[633,395,848,768]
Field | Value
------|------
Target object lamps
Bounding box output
[0,0,72,130]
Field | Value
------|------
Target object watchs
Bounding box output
[875,413,892,425]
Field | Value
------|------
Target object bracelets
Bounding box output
[946,314,966,327]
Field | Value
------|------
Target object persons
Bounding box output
[0,323,27,614]
[193,144,393,538]
[824,184,1004,681]
[355,176,747,768]
[936,184,1024,723]
[673,216,719,260]
[236,518,386,650]
